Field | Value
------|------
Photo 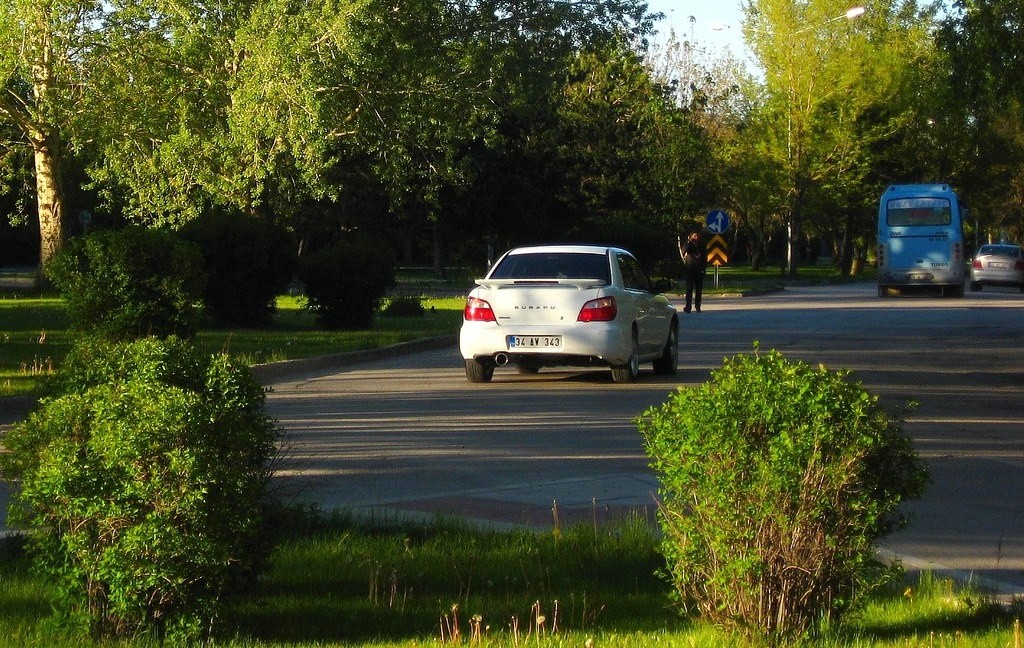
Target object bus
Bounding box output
[877,183,965,297]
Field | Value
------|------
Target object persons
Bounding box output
[680,232,706,313]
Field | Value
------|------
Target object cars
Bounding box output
[968,244,1024,293]
[458,243,683,383]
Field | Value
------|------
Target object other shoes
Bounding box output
[696,306,700,312]
[684,307,691,312]
